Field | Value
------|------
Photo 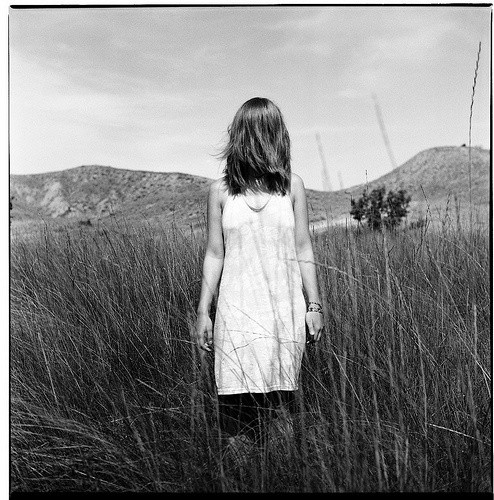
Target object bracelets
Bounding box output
[307,301,323,314]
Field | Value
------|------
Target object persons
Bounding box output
[195,97,326,396]
